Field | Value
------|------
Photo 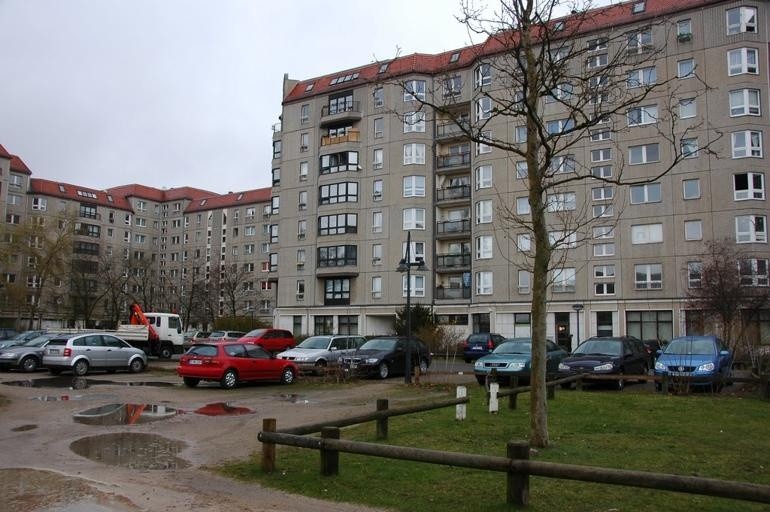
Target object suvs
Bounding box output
[463,332,507,364]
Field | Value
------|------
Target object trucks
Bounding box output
[46,312,185,360]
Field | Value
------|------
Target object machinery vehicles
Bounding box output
[121,401,179,427]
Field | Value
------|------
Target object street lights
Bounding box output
[394,230,433,387]
[38,310,44,329]
[572,304,585,346]
[249,305,255,330]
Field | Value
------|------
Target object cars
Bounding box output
[641,339,669,369]
[338,336,432,381]
[0,327,68,374]
[236,328,296,354]
[183,330,248,351]
[558,335,651,391]
[474,337,569,387]
[275,333,368,377]
[42,332,148,376]
[176,340,298,390]
[654,335,734,393]
